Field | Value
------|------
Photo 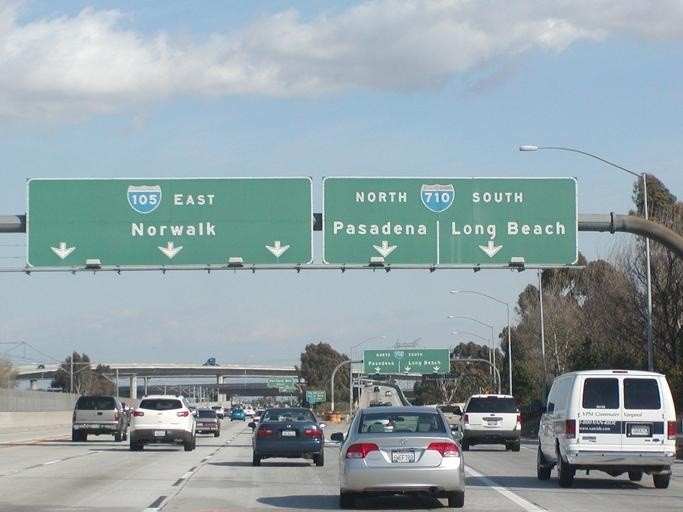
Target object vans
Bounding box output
[452,393,523,452]
[72,395,128,442]
[536,368,680,491]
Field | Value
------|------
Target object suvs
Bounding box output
[129,394,197,451]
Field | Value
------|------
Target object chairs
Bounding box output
[146,403,156,409]
[368,423,435,433]
[269,416,305,421]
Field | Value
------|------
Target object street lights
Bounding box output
[450,290,512,396]
[448,314,496,394]
[518,144,655,372]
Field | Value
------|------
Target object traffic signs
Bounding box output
[363,348,451,377]
[320,173,580,269]
[23,176,315,268]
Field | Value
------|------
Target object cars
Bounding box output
[231,408,245,421]
[248,406,328,466]
[194,408,221,438]
[330,405,466,508]
